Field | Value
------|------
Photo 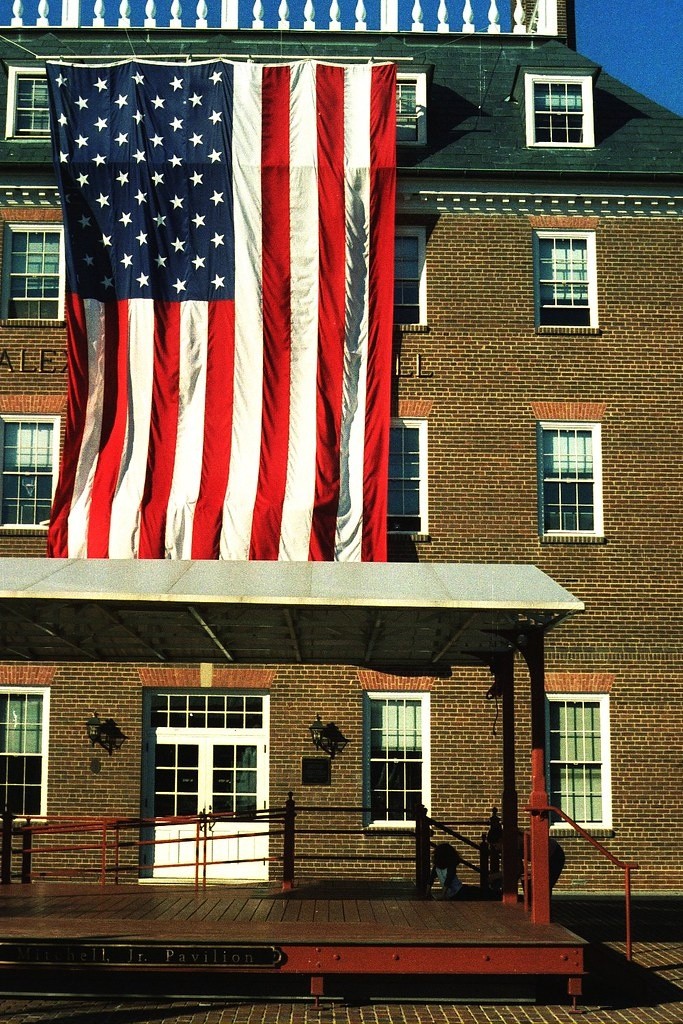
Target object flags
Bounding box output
[46,58,399,563]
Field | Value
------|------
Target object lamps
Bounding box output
[84,709,105,748]
[308,712,326,751]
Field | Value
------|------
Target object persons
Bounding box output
[425,828,565,924]
